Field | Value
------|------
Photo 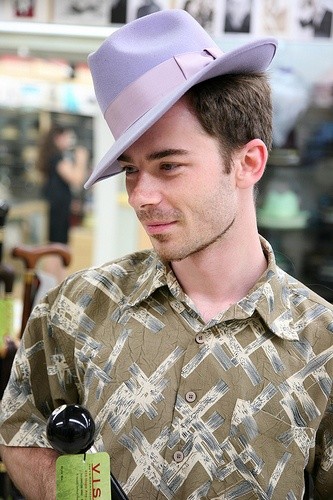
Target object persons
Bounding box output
[38,123,89,243]
[0,7,333,500]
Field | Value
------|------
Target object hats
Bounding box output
[83,9,279,191]
